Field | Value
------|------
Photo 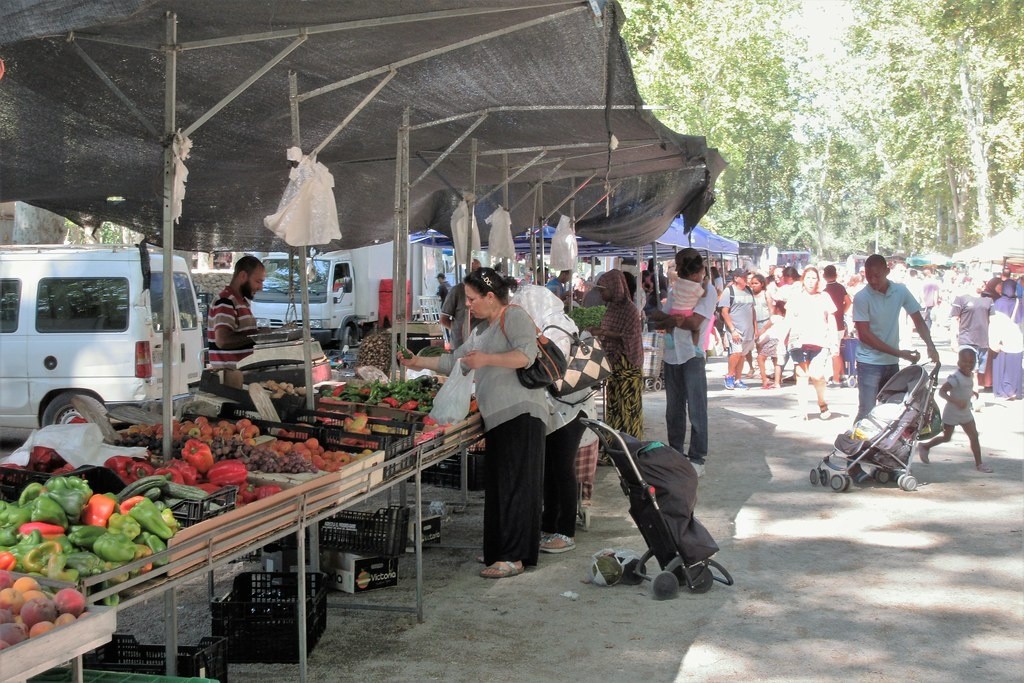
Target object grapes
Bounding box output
[115,431,319,472]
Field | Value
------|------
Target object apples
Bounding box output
[127,415,260,446]
[259,439,373,472]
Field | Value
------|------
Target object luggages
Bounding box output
[579,416,734,599]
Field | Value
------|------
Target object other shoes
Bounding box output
[796,410,809,421]
[818,403,831,419]
[769,383,781,389]
[976,463,993,473]
[918,443,929,464]
[746,370,756,378]
[761,379,772,388]
[689,462,705,478]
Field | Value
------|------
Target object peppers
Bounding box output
[4,438,285,507]
[319,374,479,435]
[396,343,412,359]
[0,476,178,606]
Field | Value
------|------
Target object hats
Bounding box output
[732,268,751,277]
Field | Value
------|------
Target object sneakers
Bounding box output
[723,374,735,390]
[734,379,748,389]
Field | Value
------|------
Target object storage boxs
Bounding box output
[83,634,229,683]
[323,551,400,594]
[210,572,327,663]
[404,515,441,547]
[199,369,484,487]
[20,668,220,683]
[263,505,410,574]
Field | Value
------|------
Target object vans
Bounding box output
[0,244,206,442]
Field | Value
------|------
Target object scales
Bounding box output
[237,328,325,371]
[386,322,445,341]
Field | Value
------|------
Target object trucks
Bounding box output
[249,238,443,350]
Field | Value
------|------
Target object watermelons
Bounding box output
[416,346,449,356]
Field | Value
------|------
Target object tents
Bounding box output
[409,213,739,314]
[953,225,1024,273]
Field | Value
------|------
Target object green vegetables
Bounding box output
[567,304,607,328]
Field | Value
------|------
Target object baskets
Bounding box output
[0,456,239,529]
[178,395,488,494]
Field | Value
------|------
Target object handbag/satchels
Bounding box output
[501,304,568,390]
[539,325,613,408]
[428,358,476,425]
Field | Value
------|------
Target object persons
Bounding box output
[531,267,549,285]
[588,269,644,464]
[206,256,275,364]
[894,261,1024,401]
[511,283,594,552]
[918,348,993,473]
[718,264,869,423]
[435,273,452,308]
[852,254,940,426]
[652,248,718,477]
[440,258,487,355]
[546,270,570,301]
[396,267,545,577]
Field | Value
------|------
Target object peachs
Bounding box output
[0,569,93,651]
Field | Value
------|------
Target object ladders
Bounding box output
[419,297,449,345]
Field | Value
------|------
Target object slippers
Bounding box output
[539,533,576,554]
[479,560,524,578]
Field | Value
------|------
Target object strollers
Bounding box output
[810,361,942,492]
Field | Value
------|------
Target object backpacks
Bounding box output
[714,285,734,337]
